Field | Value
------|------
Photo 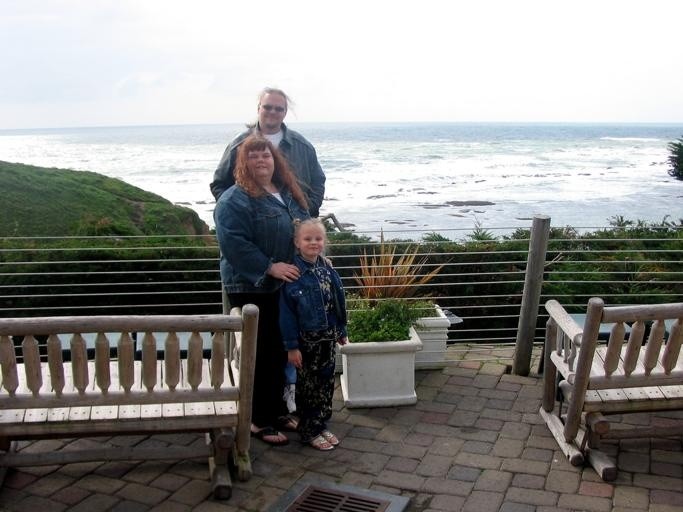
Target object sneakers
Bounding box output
[283,384,297,414]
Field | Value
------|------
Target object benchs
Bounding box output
[538,298,682,481]
[0,303,259,502]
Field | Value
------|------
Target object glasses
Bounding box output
[260,105,284,112]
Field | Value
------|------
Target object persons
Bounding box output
[213,136,333,446]
[280,218,347,451]
[210,89,326,414]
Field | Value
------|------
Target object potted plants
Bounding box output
[333,228,450,370]
[335,296,422,407]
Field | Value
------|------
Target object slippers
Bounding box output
[252,428,288,445]
[310,431,338,450]
[279,416,299,431]
